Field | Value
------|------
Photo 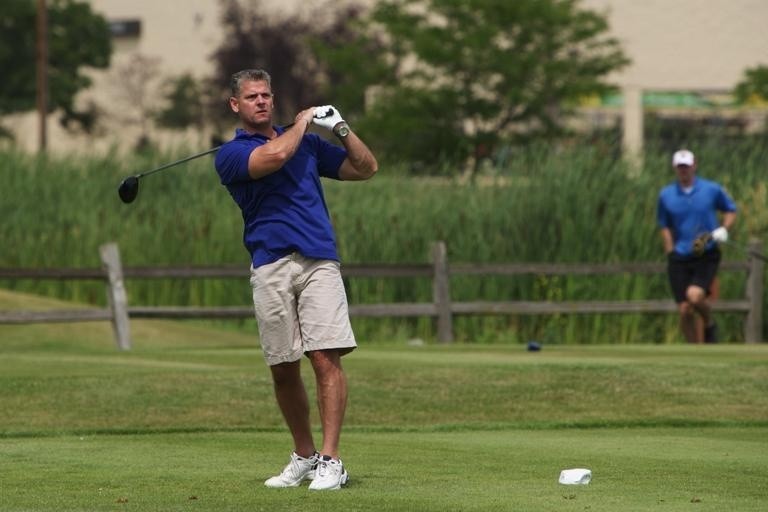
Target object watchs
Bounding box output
[335,124,351,139]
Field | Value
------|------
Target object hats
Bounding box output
[672,149,694,168]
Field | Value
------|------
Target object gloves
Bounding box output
[712,227,728,243]
[311,104,346,132]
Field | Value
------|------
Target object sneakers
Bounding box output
[704,320,719,343]
[309,453,349,492]
[263,451,320,489]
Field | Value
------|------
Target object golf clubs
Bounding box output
[118,108,334,203]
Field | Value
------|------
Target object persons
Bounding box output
[655,148,738,345]
[212,66,379,491]
